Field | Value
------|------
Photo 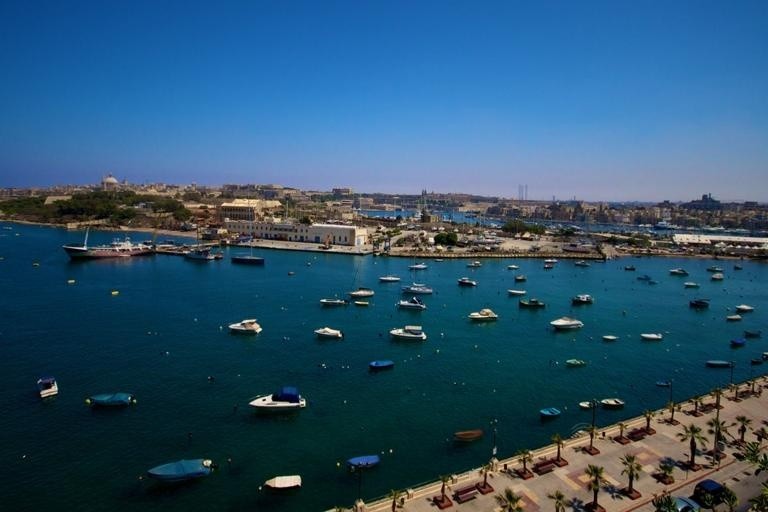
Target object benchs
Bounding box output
[533,460,555,474]
[628,429,646,440]
[454,485,479,502]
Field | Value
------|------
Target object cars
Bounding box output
[675,479,726,512]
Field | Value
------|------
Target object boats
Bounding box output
[63,184,264,266]
[354,301,369,306]
[743,329,761,337]
[706,359,732,368]
[656,381,672,387]
[684,282,700,288]
[263,476,302,489]
[729,337,747,345]
[602,336,617,341]
[457,276,477,287]
[507,264,520,270]
[550,317,585,329]
[600,399,625,406]
[726,314,742,320]
[467,308,501,321]
[518,298,545,307]
[390,326,426,341]
[625,265,636,271]
[35,373,59,400]
[408,263,428,270]
[320,298,346,306]
[350,287,375,298]
[85,392,137,409]
[379,276,401,282]
[247,386,306,410]
[668,268,689,276]
[565,359,586,367]
[571,294,594,304]
[712,273,724,280]
[706,266,724,272]
[313,327,344,339]
[401,283,434,294]
[539,407,560,417]
[397,296,426,311]
[750,352,768,364]
[454,430,483,441]
[735,304,754,312]
[508,289,527,296]
[229,317,263,335]
[543,259,558,269]
[579,401,595,409]
[514,274,528,280]
[639,333,663,340]
[466,260,482,267]
[369,360,395,369]
[575,261,591,266]
[146,458,216,485]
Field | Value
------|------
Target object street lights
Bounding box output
[350,461,372,502]
